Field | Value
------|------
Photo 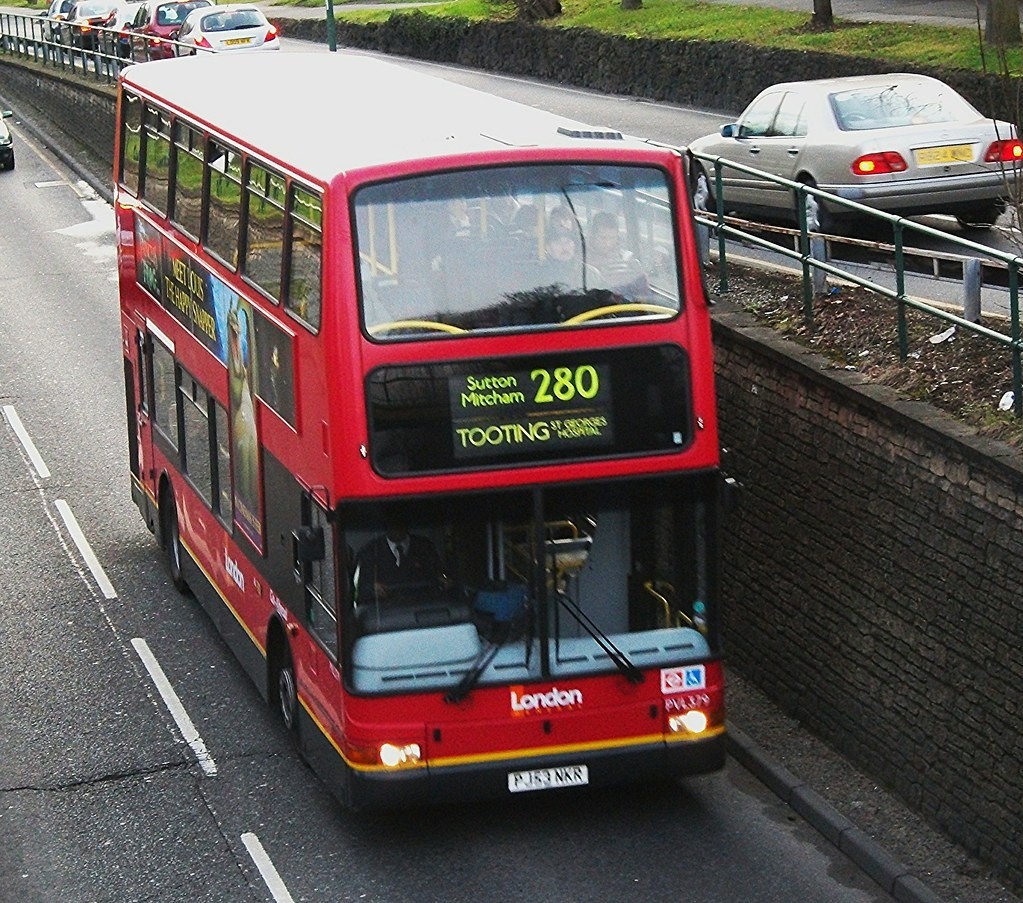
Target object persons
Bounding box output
[591,211,651,303]
[355,529,445,607]
[517,205,606,294]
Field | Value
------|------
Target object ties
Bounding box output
[395,545,405,567]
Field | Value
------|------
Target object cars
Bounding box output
[685,74,1023,258]
[0,105,16,174]
[34,0,282,63]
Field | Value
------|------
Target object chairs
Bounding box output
[157,11,166,22]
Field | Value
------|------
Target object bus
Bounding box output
[113,49,727,816]
